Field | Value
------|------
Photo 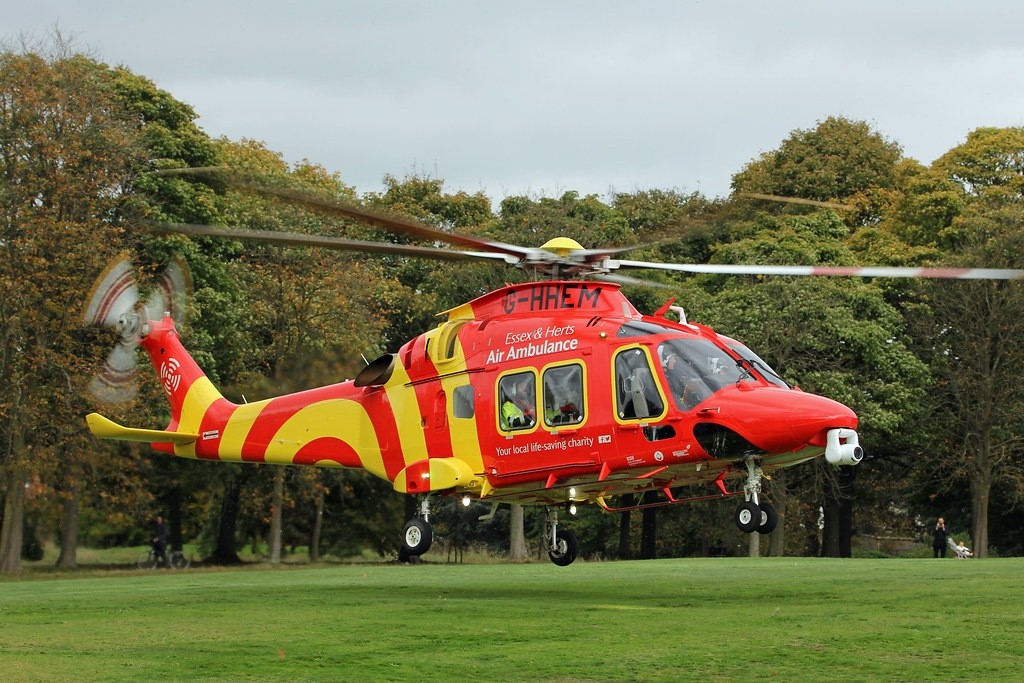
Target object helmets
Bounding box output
[657,344,676,368]
[510,379,533,399]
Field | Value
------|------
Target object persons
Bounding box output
[702,356,729,387]
[957,541,974,555]
[933,517,948,558]
[644,344,689,417]
[500,377,575,430]
[152,516,169,571]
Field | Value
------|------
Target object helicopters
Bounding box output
[79,169,1024,568]
